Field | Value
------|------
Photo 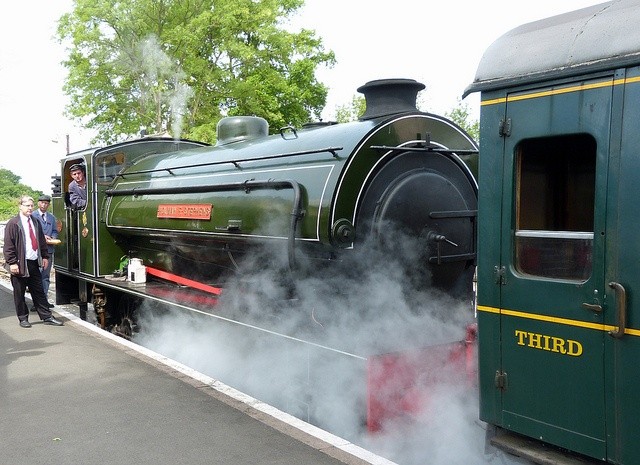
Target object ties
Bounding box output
[42,214,44,221]
[28,218,38,251]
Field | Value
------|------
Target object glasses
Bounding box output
[22,205,33,207]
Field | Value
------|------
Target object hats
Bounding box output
[38,195,50,202]
[69,164,83,171]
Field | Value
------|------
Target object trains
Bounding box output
[50,0,640,465]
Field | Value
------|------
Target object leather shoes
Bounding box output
[49,304,54,308]
[20,320,31,327]
[30,306,37,311]
[44,316,63,326]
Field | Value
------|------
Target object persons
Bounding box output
[3,195,63,328]
[68,163,87,210]
[30,195,58,312]
